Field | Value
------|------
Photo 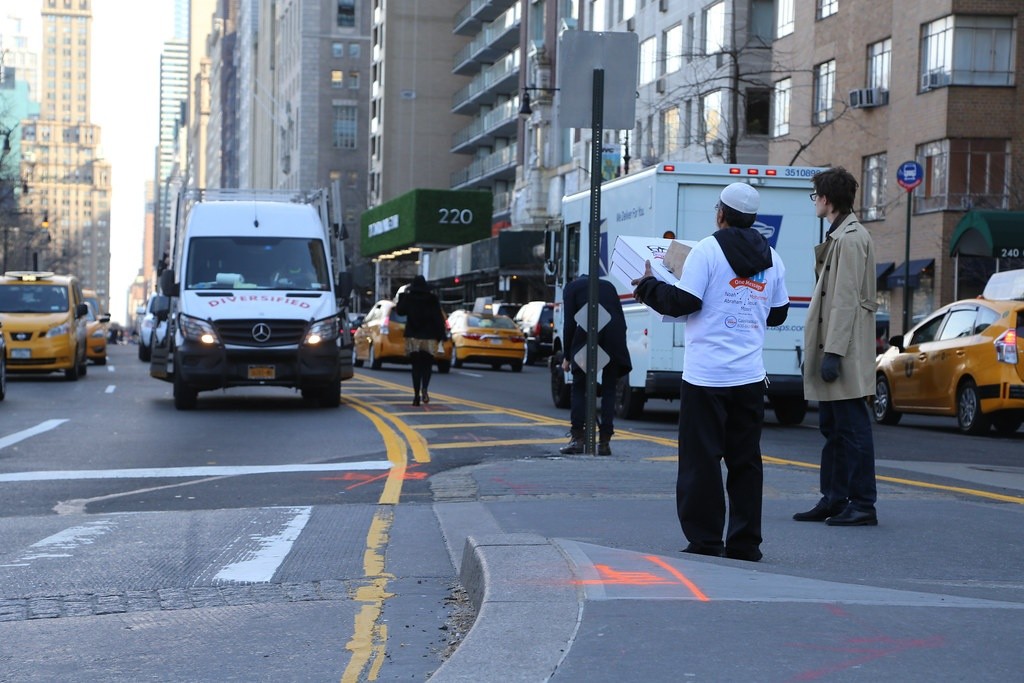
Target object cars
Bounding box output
[869,294,1024,437]
[59,288,173,381]
[445,309,529,373]
[352,297,454,374]
[0,270,89,382]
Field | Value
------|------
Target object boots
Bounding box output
[598,429,615,455]
[559,420,586,454]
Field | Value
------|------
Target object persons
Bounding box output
[793,167,879,525]
[111,330,123,344]
[559,272,633,455]
[395,275,446,405]
[631,183,789,561]
[270,251,318,287]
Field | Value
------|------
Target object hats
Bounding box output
[720,182,760,214]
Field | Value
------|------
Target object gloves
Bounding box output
[801,362,804,376]
[820,352,840,383]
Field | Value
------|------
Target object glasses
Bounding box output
[810,193,819,202]
[715,203,723,211]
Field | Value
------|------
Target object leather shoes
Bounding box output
[825,504,878,527]
[793,500,847,523]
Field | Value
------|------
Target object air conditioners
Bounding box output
[921,72,940,90]
[850,86,886,107]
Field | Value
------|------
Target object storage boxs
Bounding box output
[609,232,701,295]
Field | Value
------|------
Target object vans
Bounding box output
[543,159,833,426]
[159,200,352,410]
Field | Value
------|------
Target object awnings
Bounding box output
[888,259,933,288]
[876,262,894,278]
[949,209,1024,301]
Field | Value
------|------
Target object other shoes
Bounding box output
[413,396,420,406]
[422,394,429,403]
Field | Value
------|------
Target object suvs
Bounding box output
[513,300,554,365]
